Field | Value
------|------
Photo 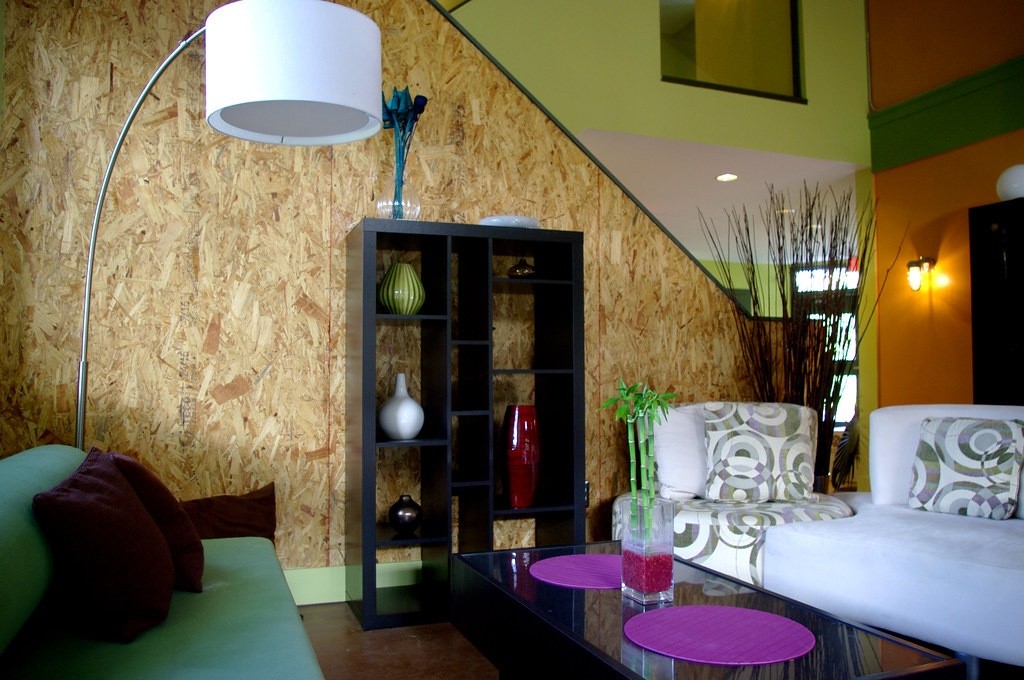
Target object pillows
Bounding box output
[908,416,1024,522]
[702,401,820,503]
[179,482,305,623]
[31,447,176,644]
[107,452,205,594]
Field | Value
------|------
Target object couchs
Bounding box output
[642,404,1024,680]
[0,443,328,680]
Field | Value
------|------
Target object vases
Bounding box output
[507,258,538,280]
[376,372,425,441]
[377,167,421,221]
[498,405,542,509]
[377,263,426,315]
[388,495,424,533]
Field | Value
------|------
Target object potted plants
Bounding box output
[591,375,674,606]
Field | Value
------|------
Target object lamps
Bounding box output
[74,0,384,451]
[996,164,1024,201]
[906,257,936,292]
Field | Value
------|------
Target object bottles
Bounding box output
[378,263,426,315]
[388,494,423,535]
[378,374,425,440]
[506,259,537,279]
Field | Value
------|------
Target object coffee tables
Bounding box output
[447,540,968,680]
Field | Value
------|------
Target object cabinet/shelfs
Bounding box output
[343,216,587,633]
[967,197,1024,406]
